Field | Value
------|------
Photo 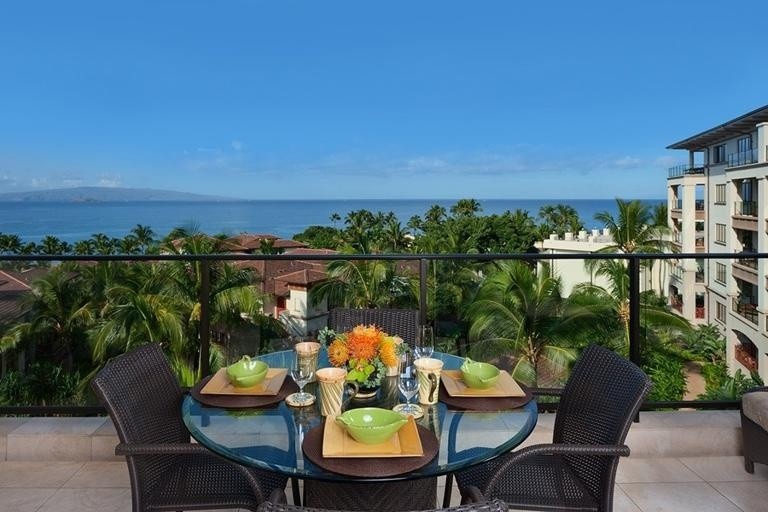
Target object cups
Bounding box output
[414,358,444,404]
[315,367,358,416]
[296,342,320,383]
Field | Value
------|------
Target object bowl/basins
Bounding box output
[226,355,269,386]
[461,357,500,389]
[335,407,407,444]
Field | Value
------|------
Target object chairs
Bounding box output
[91,347,302,511]
[325,308,422,349]
[256,473,509,511]
[443,343,653,512]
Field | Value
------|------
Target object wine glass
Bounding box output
[415,325,434,357]
[286,360,316,407]
[393,356,424,418]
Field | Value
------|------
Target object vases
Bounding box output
[346,387,380,403]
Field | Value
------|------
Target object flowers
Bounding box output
[316,324,414,388]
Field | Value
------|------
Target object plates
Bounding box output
[322,415,424,458]
[441,368,526,398]
[200,368,288,395]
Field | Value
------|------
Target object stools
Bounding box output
[740,386,768,473]
